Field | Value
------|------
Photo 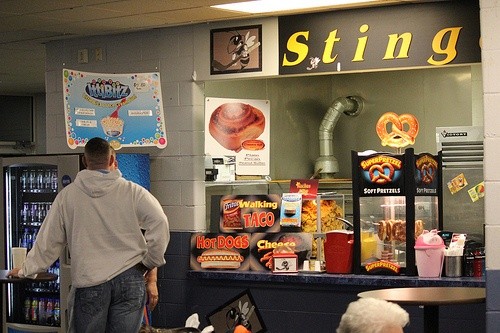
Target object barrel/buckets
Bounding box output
[444,256,462,277]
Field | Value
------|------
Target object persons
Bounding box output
[5,137,170,333]
[336,297,410,333]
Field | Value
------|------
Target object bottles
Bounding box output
[464,249,474,277]
[24,297,31,320]
[48,258,60,292]
[47,299,60,325]
[32,229,38,247]
[24,281,47,292]
[21,202,53,222]
[37,170,58,190]
[31,298,47,321]
[474,248,482,277]
[21,170,36,189]
[21,228,32,256]
[310,257,317,271]
[481,248,486,277]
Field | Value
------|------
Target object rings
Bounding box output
[154,298,157,300]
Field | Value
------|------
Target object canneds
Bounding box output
[22,202,52,223]
[21,168,58,192]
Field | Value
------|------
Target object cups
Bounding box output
[13,248,27,269]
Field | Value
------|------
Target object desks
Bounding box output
[357,286,485,333]
[0,270,58,284]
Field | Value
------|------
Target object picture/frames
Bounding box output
[209,23,264,75]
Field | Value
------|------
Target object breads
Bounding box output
[376,112,420,147]
[208,101,266,153]
[368,162,394,183]
[377,220,424,242]
[420,162,435,184]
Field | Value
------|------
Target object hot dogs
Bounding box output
[197,249,244,268]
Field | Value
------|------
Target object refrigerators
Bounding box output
[0,153,152,333]
